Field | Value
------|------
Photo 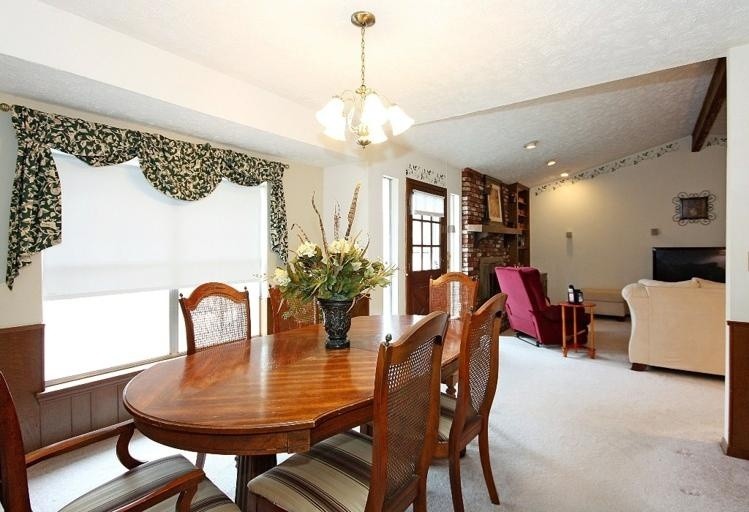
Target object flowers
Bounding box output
[268,181,407,321]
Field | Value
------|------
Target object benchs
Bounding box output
[581,287,628,322]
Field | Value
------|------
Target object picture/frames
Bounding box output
[483,173,507,226]
[669,190,717,228]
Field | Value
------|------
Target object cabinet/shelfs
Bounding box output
[507,183,530,250]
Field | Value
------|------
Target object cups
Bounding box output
[576,292,583,303]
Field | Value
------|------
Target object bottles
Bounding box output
[568,289,575,303]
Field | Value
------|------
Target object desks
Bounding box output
[124,313,462,512]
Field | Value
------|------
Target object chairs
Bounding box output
[620,275,727,376]
[497,264,591,349]
[0,370,239,512]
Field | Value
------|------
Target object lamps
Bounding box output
[315,8,414,149]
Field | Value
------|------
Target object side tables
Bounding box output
[560,301,597,359]
